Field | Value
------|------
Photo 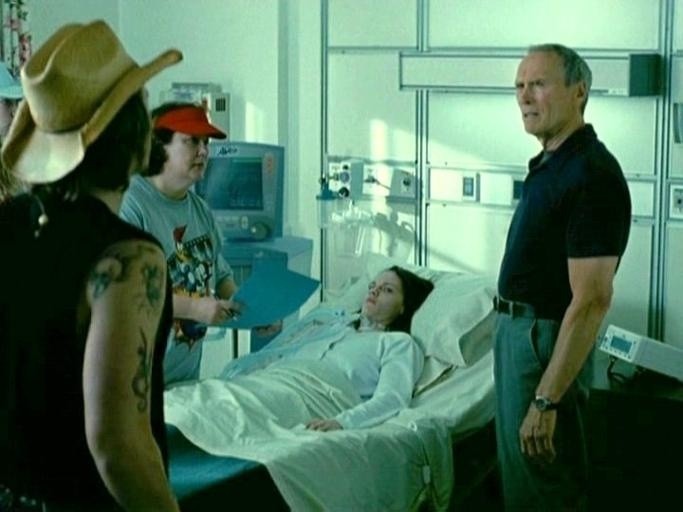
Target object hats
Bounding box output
[1,19,183,184]
[152,107,227,140]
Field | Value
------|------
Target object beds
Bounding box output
[154,282,495,512]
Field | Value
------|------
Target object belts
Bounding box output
[492,295,563,320]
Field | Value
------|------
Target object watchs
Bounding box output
[528,391,559,412]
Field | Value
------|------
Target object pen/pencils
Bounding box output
[209,289,237,321]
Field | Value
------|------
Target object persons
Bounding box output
[492,43,632,511]
[1,18,186,512]
[116,99,281,391]
[0,60,24,139]
[159,264,440,452]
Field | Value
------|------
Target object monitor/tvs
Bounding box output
[189,140,285,241]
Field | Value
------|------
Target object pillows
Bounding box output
[332,252,496,367]
